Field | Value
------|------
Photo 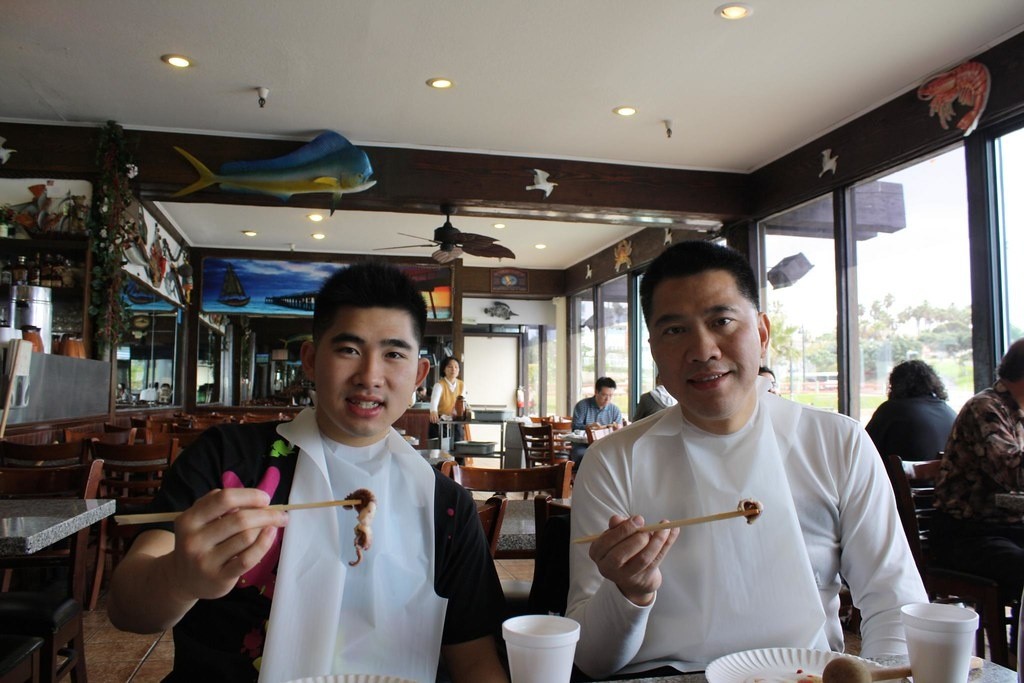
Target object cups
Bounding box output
[502,615,580,683]
[901,603,979,683]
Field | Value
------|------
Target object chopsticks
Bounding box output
[571,509,759,544]
[114,500,361,525]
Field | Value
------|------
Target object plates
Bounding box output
[286,674,416,683]
[705,647,913,683]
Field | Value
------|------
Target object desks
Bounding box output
[0,498,115,558]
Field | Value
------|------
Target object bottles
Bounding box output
[622,419,627,426]
[455,396,466,420]
[559,417,562,422]
[22,326,44,353]
[51,334,86,359]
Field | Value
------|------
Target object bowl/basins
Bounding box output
[574,430,585,435]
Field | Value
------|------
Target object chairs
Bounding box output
[0,410,1022,683]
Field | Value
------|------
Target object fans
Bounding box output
[371,204,514,263]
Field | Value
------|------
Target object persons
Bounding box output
[566,240,931,683]
[429,357,472,469]
[117,383,137,404]
[416,386,427,402]
[109,260,512,683]
[865,338,1024,665]
[152,382,171,404]
[569,366,779,478]
